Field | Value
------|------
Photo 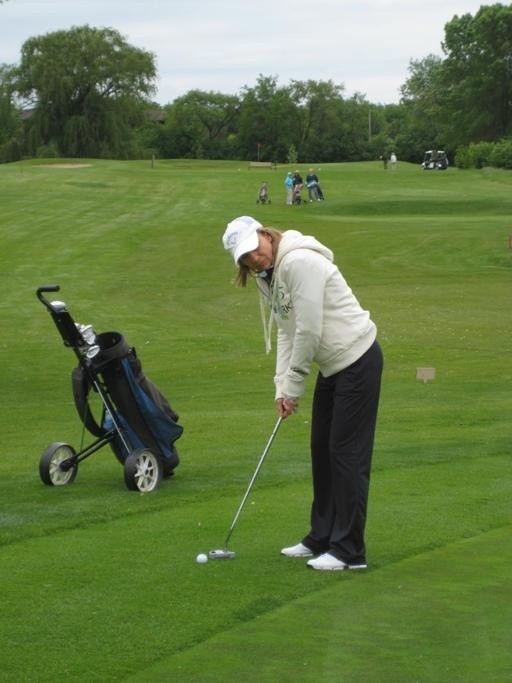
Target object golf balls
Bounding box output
[196,553,208,564]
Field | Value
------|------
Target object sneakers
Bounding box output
[281,543,314,558]
[307,552,367,571]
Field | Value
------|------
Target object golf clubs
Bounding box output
[209,414,284,560]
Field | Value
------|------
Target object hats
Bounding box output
[222,215,263,268]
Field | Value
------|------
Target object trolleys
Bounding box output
[257,180,273,205]
[291,183,305,205]
[29,280,180,493]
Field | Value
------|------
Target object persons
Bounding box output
[305,168,322,203]
[221,215,385,570]
[292,170,304,204]
[390,151,397,171]
[283,171,294,205]
[379,153,389,169]
